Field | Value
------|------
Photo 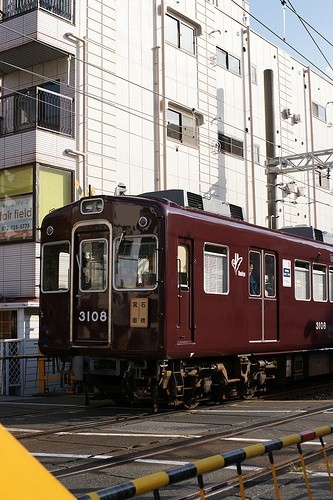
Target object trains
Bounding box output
[39,183,333,411]
